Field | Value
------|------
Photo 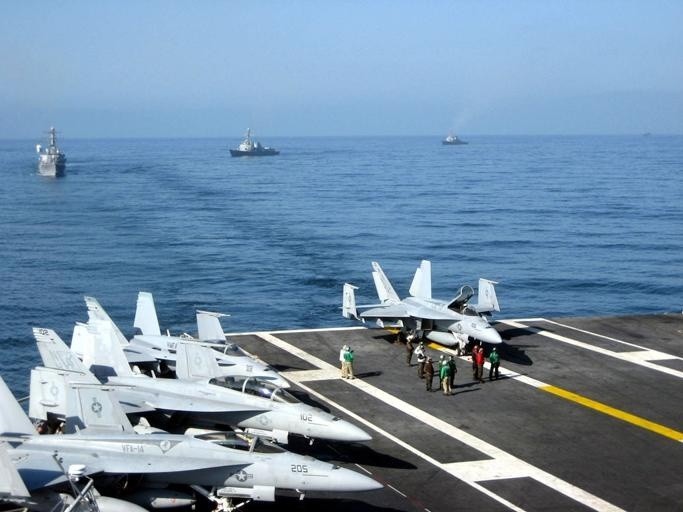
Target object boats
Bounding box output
[443,135,468,144]
[36,127,65,177]
[229,128,280,157]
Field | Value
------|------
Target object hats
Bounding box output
[493,348,496,351]
[420,342,424,349]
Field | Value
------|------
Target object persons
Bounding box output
[476,348,485,381]
[472,345,479,380]
[405,339,414,367]
[489,347,500,381]
[344,346,354,379]
[339,344,348,378]
[413,341,457,395]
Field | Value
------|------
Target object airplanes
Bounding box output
[342,259,503,356]
